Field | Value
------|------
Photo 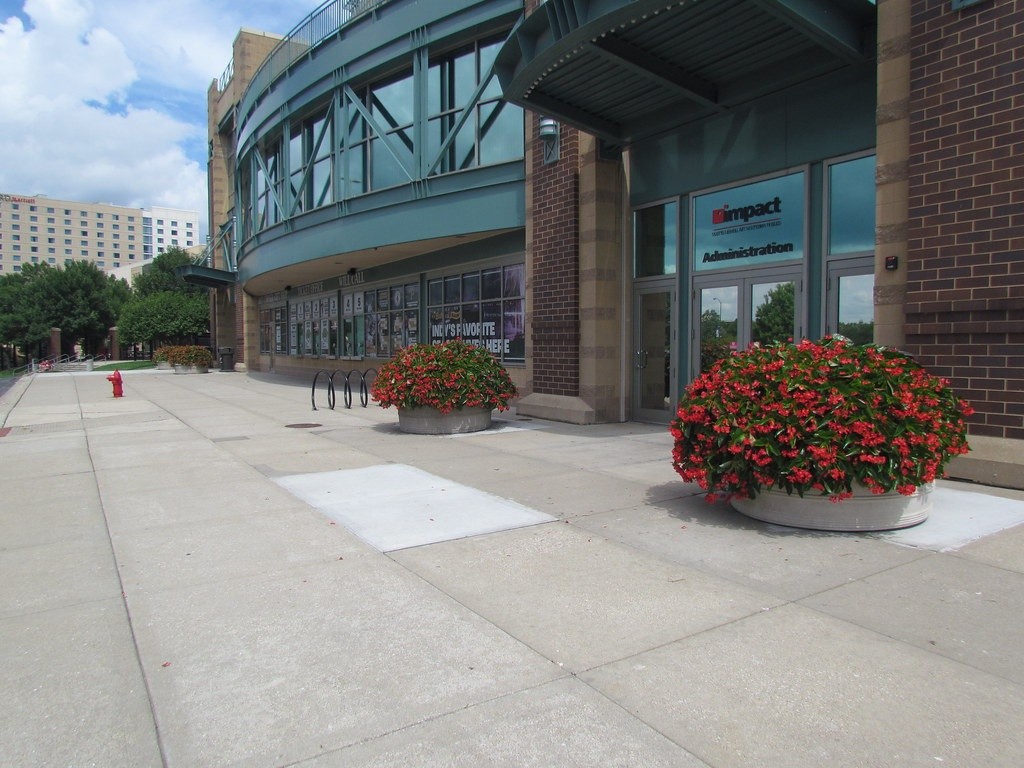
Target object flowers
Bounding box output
[669,332,975,508]
[664,341,731,399]
[151,344,213,369]
[370,337,519,415]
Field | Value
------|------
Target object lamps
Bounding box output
[538,114,560,166]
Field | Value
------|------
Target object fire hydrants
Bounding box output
[105,368,124,398]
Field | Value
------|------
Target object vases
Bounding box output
[398,404,492,435]
[727,471,936,531]
[155,361,208,374]
